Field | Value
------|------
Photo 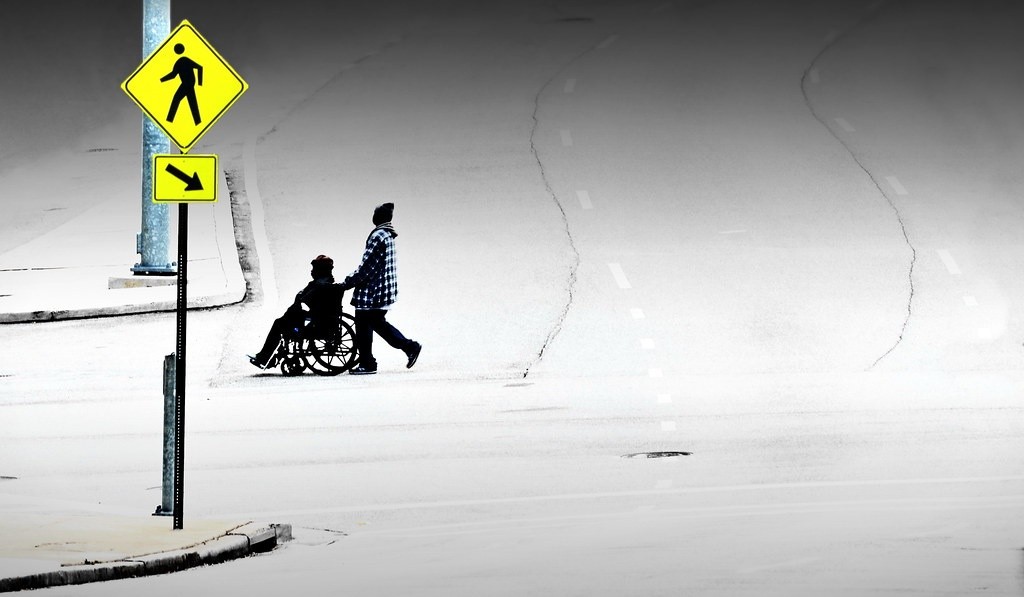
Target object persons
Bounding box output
[340,200,422,375]
[257,255,339,368]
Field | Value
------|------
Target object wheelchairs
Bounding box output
[249,289,362,377]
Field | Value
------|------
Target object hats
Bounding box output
[311,255,334,269]
[374,202,394,221]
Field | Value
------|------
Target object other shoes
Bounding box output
[349,362,378,374]
[406,341,422,369]
[249,358,276,370]
